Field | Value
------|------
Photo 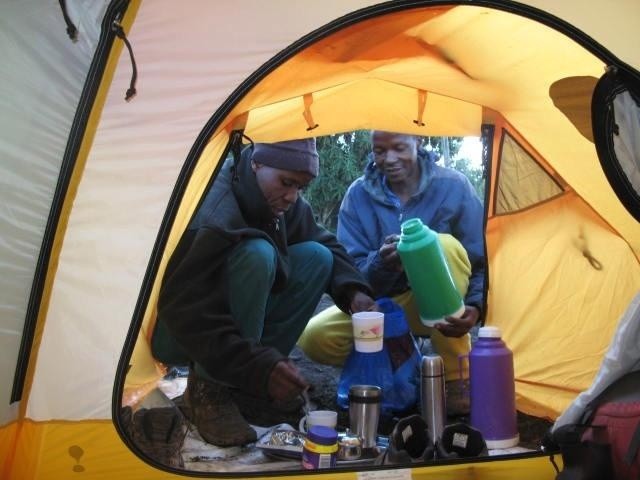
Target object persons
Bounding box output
[297,131,489,422]
[152,136,379,449]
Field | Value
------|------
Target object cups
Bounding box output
[348,385,381,457]
[352,311,385,354]
[299,411,338,431]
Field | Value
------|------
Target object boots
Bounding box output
[120,405,184,468]
[183,363,307,446]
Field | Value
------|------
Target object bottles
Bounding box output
[419,354,445,440]
[303,425,338,469]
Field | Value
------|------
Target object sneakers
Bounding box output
[375,414,488,464]
[447,381,472,419]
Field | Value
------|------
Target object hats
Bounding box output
[249,137,319,178]
